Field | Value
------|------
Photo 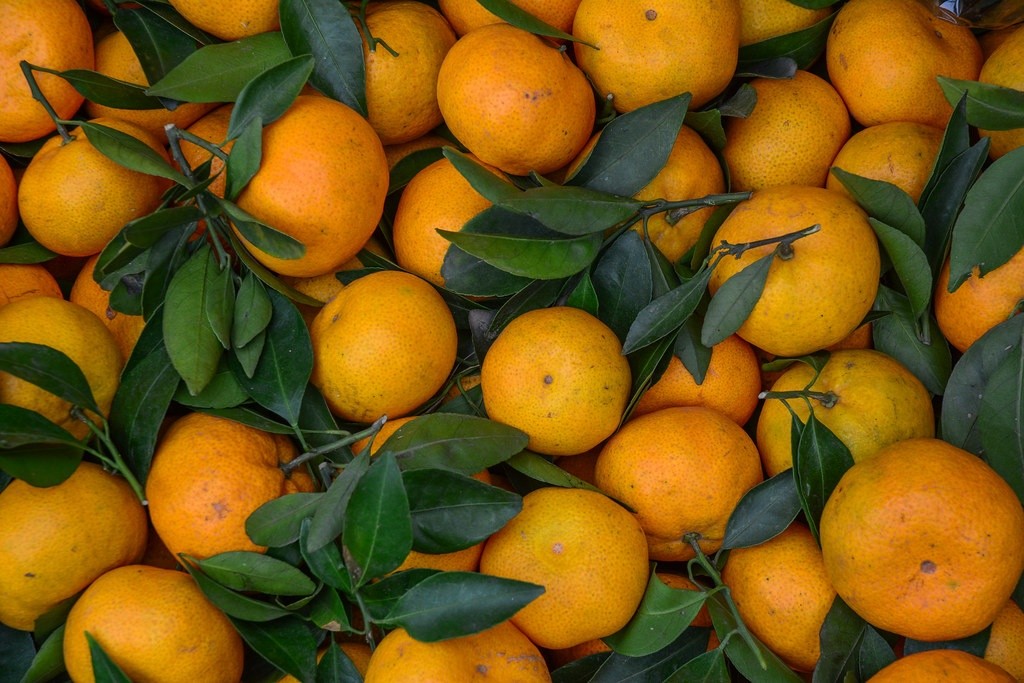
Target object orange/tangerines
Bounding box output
[0,0,1024,683]
